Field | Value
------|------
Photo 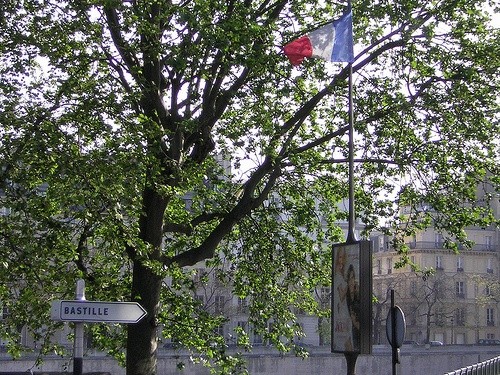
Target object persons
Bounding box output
[334,248,359,351]
[345,265,361,348]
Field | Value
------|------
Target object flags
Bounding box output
[281,5,355,64]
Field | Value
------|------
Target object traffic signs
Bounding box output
[52,298,148,323]
